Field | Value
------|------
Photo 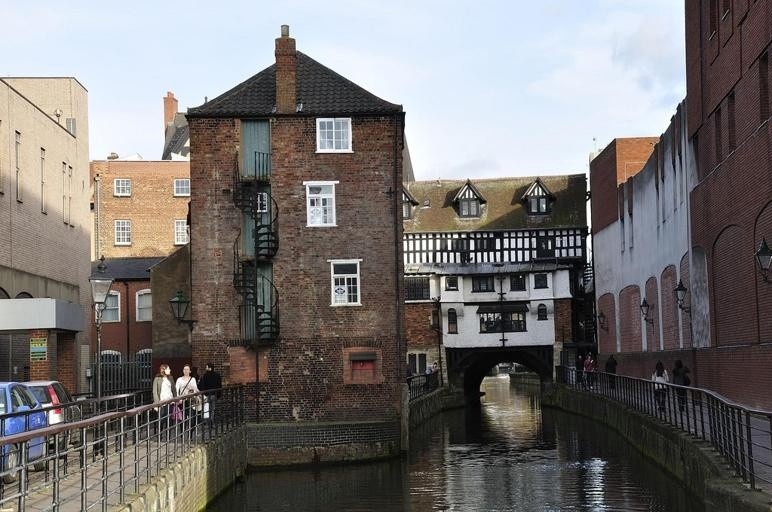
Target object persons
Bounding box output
[576,351,595,390]
[406,364,412,390]
[192,367,200,382]
[672,359,691,412]
[420,362,437,390]
[197,362,222,428]
[606,354,617,388]
[651,360,670,412]
[152,363,177,442]
[175,364,198,441]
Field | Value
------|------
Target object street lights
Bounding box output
[88,254,115,460]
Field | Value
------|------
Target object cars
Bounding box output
[19,379,82,458]
[0,381,47,484]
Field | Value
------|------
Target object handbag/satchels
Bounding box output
[172,407,182,420]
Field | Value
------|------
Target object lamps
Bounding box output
[598,309,608,332]
[753,235,772,284]
[640,297,654,327]
[168,289,198,332]
[673,278,691,316]
